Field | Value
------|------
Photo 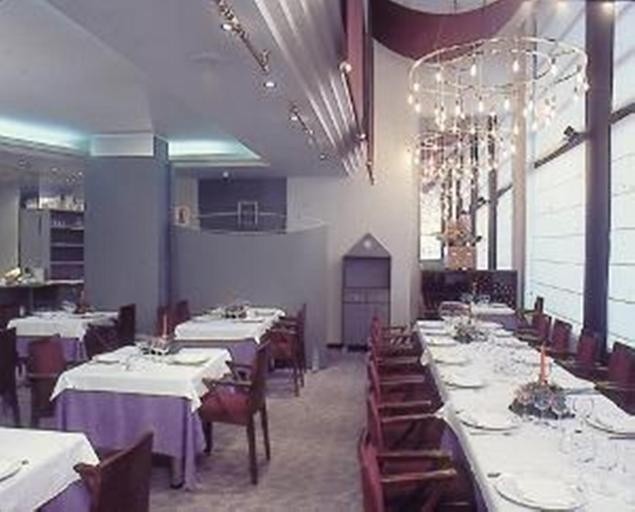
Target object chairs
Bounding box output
[157,304,175,341]
[533,319,572,360]
[556,328,601,373]
[364,351,438,399]
[365,360,443,414]
[516,296,543,326]
[599,386,635,416]
[371,323,418,355]
[199,338,274,484]
[73,431,153,511]
[573,341,635,386]
[27,333,66,429]
[373,308,414,344]
[365,336,420,373]
[174,300,189,323]
[514,313,551,344]
[0,327,22,429]
[359,429,479,512]
[259,310,304,396]
[279,304,307,389]
[83,304,135,354]
[366,389,449,451]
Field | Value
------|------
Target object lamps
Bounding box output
[408,0,589,137]
[403,125,518,224]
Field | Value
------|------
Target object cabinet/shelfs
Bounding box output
[342,233,390,355]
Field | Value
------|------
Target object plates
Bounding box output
[419,322,443,329]
[515,352,553,366]
[494,473,587,512]
[549,376,596,393]
[193,316,210,322]
[456,405,522,430]
[428,339,456,346]
[586,411,635,436]
[92,354,120,364]
[492,330,513,337]
[243,317,261,322]
[441,371,485,388]
[434,354,466,364]
[1,453,19,481]
[481,321,502,329]
[171,354,207,366]
[256,307,275,315]
[423,328,447,336]
